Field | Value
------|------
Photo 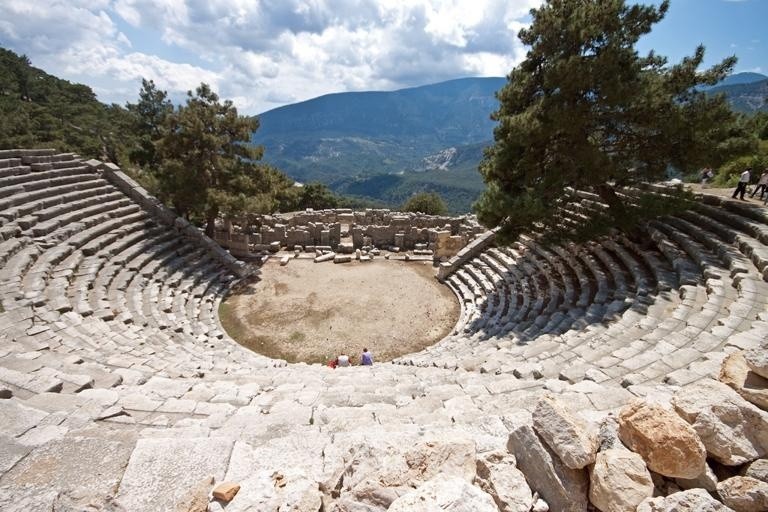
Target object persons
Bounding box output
[748,168,768,200]
[732,168,752,201]
[700,166,712,186]
[359,348,373,366]
[763,185,768,207]
[335,351,353,368]
